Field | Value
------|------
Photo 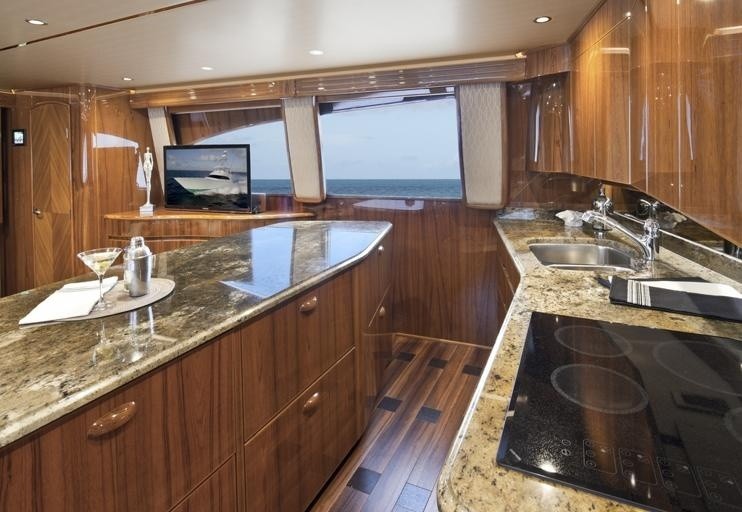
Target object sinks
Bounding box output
[523,236,642,273]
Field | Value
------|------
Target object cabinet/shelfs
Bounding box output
[239,267,357,511]
[358,230,392,441]
[0,332,237,511]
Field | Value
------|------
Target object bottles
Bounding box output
[124,236,152,298]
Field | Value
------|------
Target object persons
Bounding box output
[143,147,154,191]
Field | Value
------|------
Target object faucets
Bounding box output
[581,210,661,262]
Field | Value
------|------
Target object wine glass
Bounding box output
[77,247,122,313]
